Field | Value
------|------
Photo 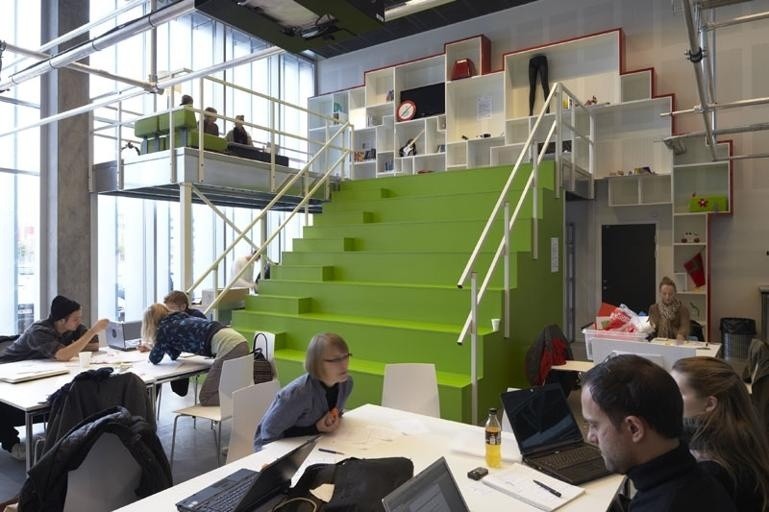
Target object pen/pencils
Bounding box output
[533,479,562,497]
[317,447,345,457]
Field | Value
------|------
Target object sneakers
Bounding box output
[0,443,26,460]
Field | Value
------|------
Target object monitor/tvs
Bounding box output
[400,82,445,120]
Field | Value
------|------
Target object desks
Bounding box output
[0,338,219,479]
[111,403,632,512]
[582,320,723,373]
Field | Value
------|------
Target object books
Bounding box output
[483,463,584,512]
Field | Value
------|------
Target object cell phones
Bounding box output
[467,466,488,481]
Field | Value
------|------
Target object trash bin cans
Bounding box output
[720,317,757,361]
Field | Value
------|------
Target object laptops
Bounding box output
[175,432,324,512]
[106,321,137,351]
[380,456,474,512]
[500,381,615,485]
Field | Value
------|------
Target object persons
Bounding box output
[577,355,736,512]
[529,56,550,116]
[0,295,108,461]
[198,107,219,135]
[180,95,192,106]
[649,276,690,345]
[141,304,249,406]
[225,115,253,146]
[670,357,769,511]
[253,334,353,451]
[146,291,206,401]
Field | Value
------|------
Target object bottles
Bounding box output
[484,408,502,468]
[568,96,572,110]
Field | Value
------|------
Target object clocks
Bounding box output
[397,100,417,122]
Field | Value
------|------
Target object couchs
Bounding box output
[135,105,228,153]
[226,142,288,166]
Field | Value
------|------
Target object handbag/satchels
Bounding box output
[254,353,273,384]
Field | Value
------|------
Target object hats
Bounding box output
[49,296,80,322]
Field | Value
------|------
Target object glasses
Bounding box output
[325,353,352,362]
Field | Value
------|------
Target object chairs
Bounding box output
[381,363,439,418]
[64,432,142,512]
[170,331,280,471]
[541,324,597,386]
[33,372,134,465]
[0,335,52,462]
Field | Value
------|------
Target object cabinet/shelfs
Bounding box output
[308,27,733,340]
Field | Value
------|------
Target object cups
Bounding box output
[491,319,501,332]
[266,147,270,153]
[79,351,93,368]
